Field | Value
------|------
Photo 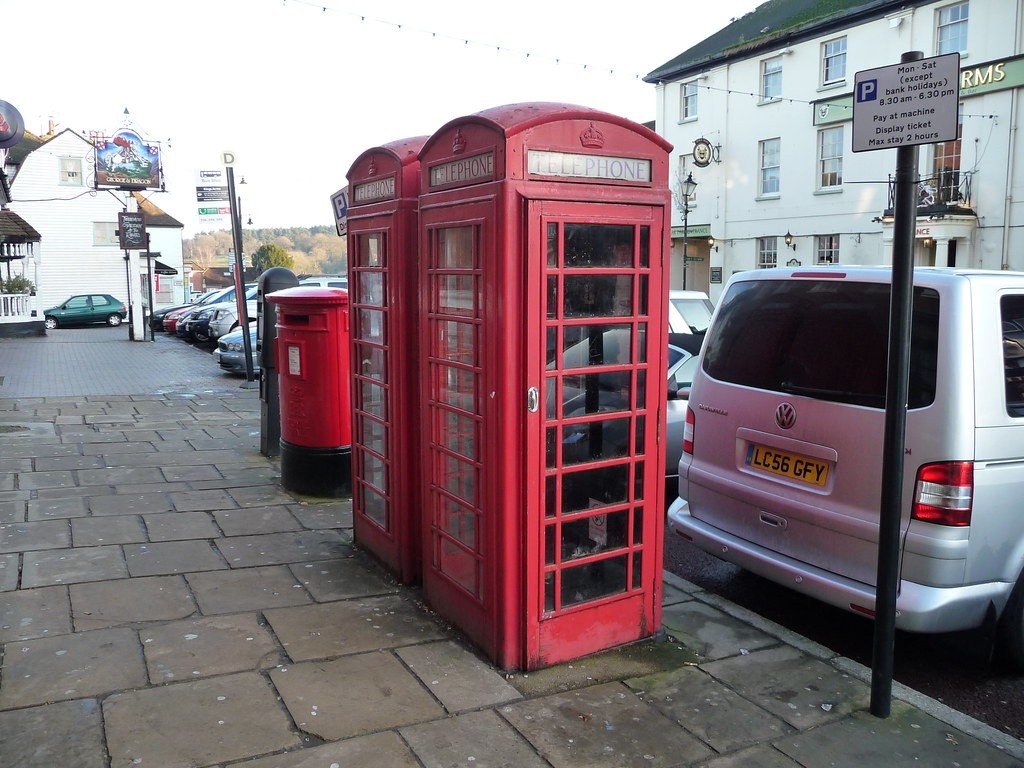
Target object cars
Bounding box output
[149,283,258,344]
[31,294,127,327]
[546,291,718,484]
[213,327,260,377]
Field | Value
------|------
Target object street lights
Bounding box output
[679,172,700,292]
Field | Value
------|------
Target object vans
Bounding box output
[666,263,1024,674]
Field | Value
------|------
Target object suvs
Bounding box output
[208,273,348,339]
